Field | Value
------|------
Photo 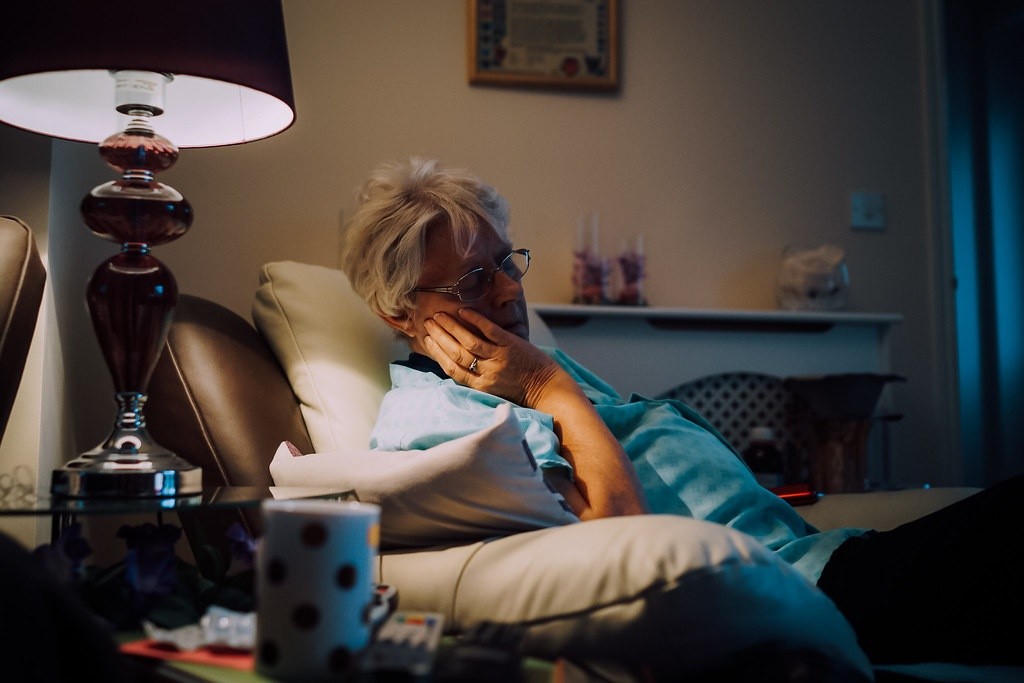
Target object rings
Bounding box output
[467,358,480,370]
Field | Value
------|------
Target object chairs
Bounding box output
[0,211,50,444]
[143,296,330,501]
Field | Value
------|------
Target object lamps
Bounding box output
[0,0,299,622]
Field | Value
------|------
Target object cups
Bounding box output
[254,500,381,679]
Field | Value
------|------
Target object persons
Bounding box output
[340,156,1023,664]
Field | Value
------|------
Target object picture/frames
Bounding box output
[466,0,625,100]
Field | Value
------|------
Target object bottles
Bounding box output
[743,427,786,497]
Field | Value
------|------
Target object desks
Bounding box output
[529,300,904,487]
[0,485,363,619]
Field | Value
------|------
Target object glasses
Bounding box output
[411,249,531,302]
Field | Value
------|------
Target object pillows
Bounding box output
[253,259,558,452]
[375,513,876,683]
[267,401,577,549]
